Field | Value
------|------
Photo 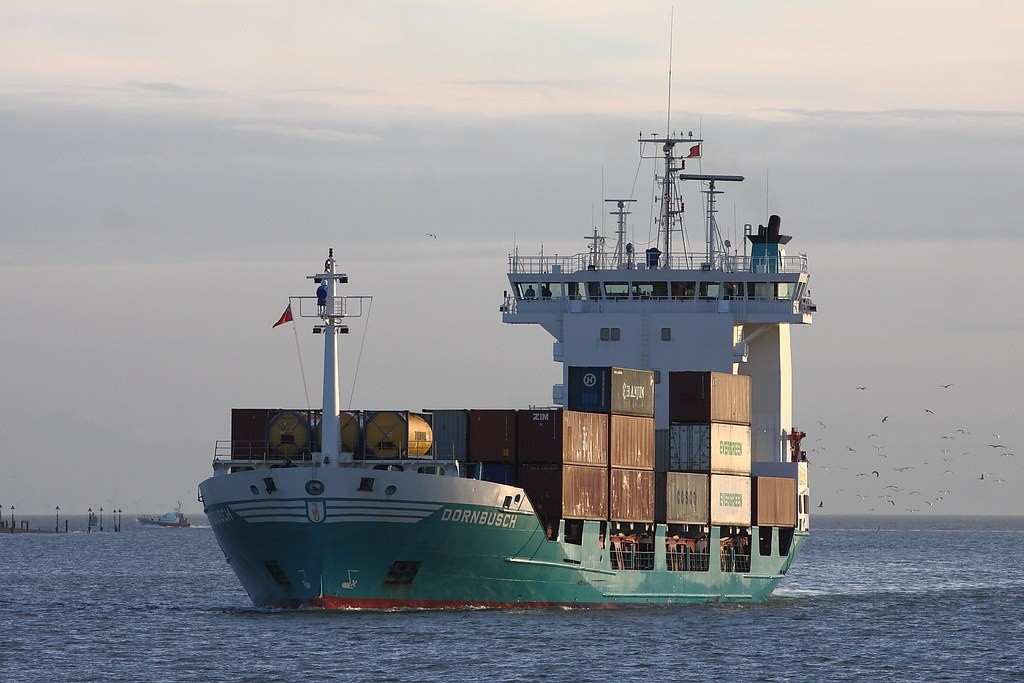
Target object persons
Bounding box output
[282,458,299,468]
[541,286,552,300]
[525,285,535,302]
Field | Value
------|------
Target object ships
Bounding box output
[197,125,817,604]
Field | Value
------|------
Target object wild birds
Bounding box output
[793,383,1015,536]
[425,232,438,240]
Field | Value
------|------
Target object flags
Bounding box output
[272,303,294,328]
[687,144,700,157]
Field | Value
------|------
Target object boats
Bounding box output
[137,501,191,528]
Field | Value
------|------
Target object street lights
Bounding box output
[11,506,15,529]
[56,505,59,532]
[87,508,92,532]
[99,508,103,530]
[113,510,118,531]
[118,509,122,531]
[0,504,2,526]
